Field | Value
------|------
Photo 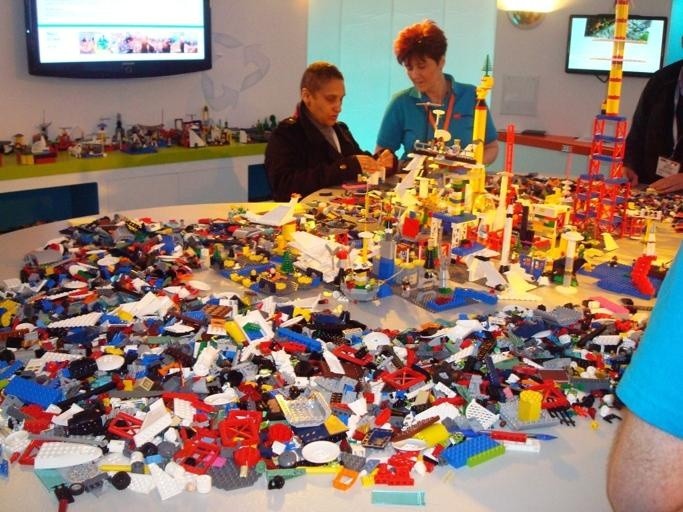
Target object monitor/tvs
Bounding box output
[565,14,668,77]
[24,0,212,79]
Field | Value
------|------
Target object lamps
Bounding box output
[495,0,576,32]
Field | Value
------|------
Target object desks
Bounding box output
[1,173,682,511]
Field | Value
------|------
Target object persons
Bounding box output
[264,59,375,201]
[620,58,682,195]
[607,240,682,512]
[372,18,501,171]
